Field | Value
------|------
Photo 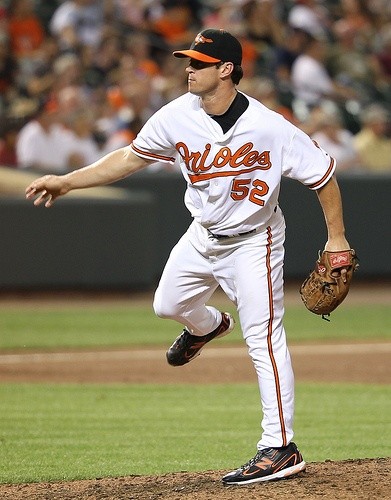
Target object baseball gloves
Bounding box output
[299,247,360,322]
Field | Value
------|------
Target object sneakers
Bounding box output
[165,313,235,366]
[220,443,305,485]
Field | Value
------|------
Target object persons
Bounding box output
[24,29,353,484]
[1,1,391,173]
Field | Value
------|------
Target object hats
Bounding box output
[172,26,242,65]
